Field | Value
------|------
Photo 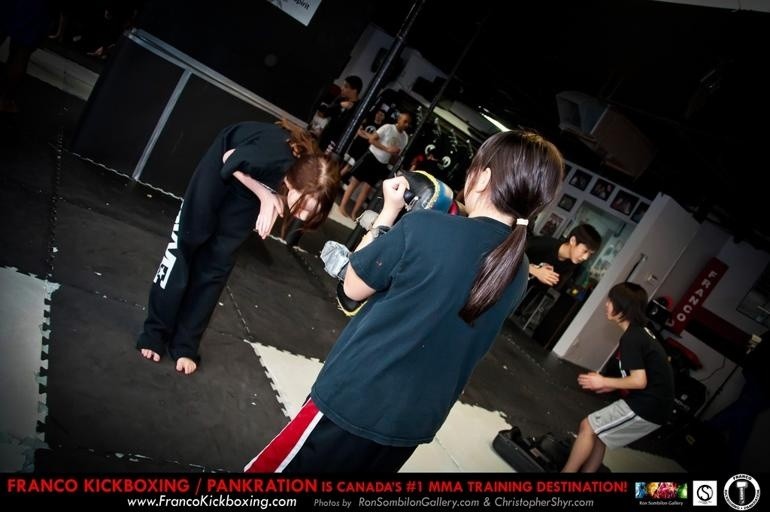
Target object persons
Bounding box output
[525,224,601,291]
[309,75,449,222]
[136,118,340,375]
[240,130,565,473]
[561,282,675,472]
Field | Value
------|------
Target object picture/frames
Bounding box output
[539,168,651,238]
